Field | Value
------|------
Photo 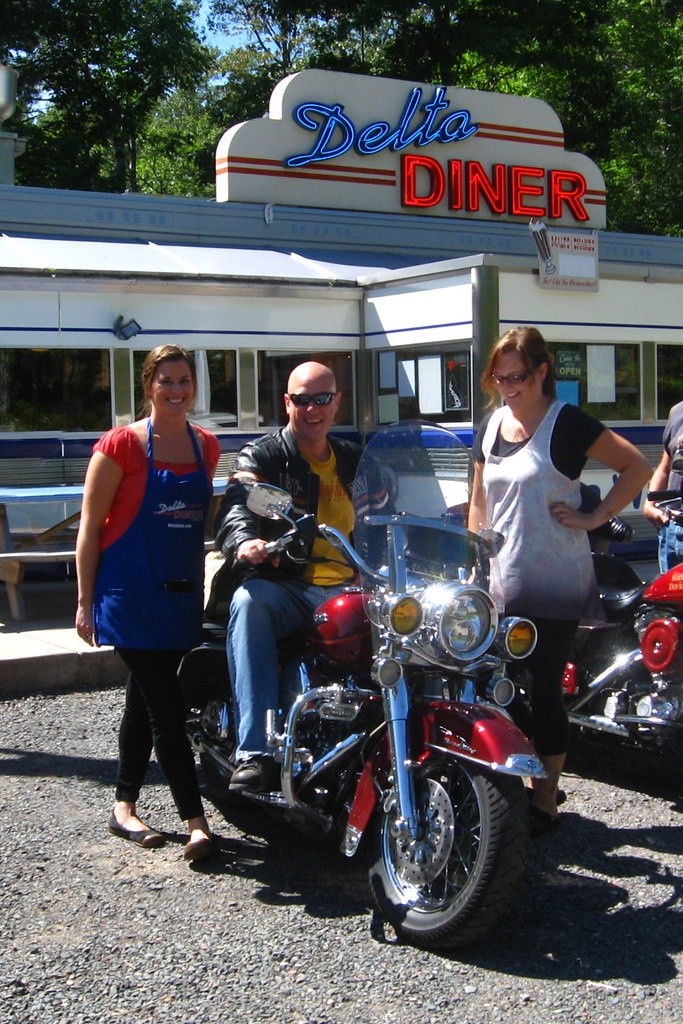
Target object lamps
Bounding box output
[113,316,142,339]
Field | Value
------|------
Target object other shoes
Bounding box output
[107,802,164,848]
[183,831,222,860]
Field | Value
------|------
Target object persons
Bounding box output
[643,401,683,574]
[466,326,653,833]
[75,344,221,861]
[206,361,401,795]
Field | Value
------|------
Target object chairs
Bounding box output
[573,482,662,614]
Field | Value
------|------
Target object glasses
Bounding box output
[289,392,336,406]
[490,370,530,384]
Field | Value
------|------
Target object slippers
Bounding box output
[525,802,561,834]
[525,786,567,806]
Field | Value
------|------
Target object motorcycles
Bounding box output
[444,489,683,758]
[166,418,549,953]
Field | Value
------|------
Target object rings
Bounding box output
[85,633,91,640]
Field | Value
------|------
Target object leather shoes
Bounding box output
[228,756,279,790]
